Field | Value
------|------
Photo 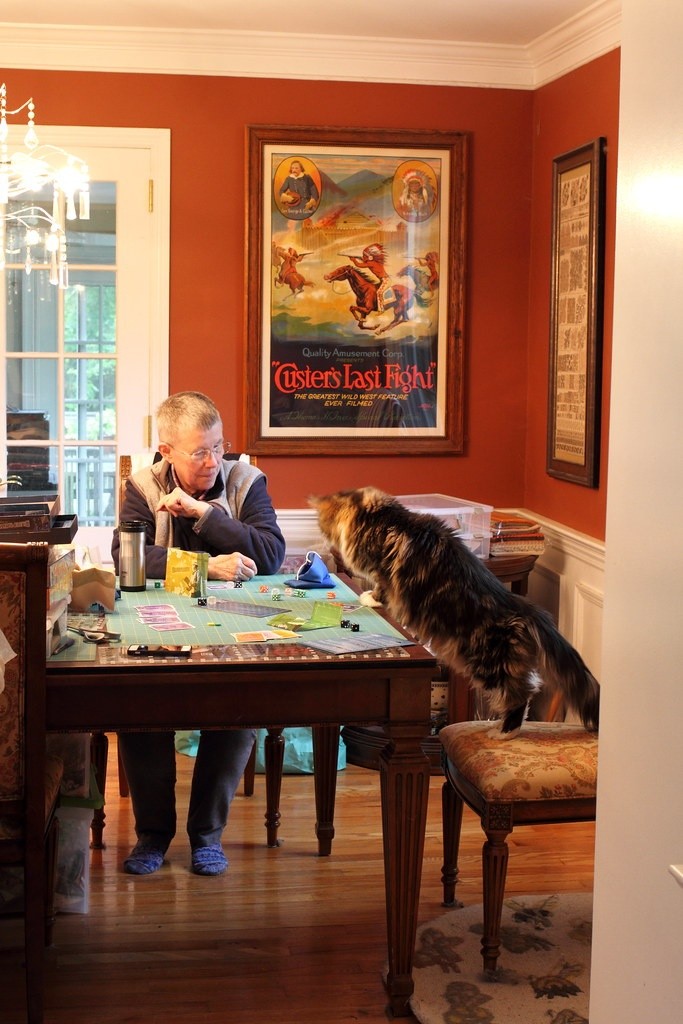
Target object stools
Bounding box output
[436,717,597,980]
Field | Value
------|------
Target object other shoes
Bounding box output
[124,840,167,874]
[191,842,228,876]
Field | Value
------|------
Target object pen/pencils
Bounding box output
[53,639,74,654]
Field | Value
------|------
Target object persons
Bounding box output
[189,560,201,598]
[111,391,286,875]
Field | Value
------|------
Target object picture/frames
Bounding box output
[242,124,467,456]
[546,135,609,489]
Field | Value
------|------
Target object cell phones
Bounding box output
[127,643,193,655]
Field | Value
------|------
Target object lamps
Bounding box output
[0,82,91,289]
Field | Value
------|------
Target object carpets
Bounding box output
[396,886,595,1023]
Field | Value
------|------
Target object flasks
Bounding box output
[119,520,146,591]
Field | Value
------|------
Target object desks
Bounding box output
[22,548,433,1024]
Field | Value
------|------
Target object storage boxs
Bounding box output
[389,490,494,563]
[0,493,79,545]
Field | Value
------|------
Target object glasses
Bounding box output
[164,441,231,465]
